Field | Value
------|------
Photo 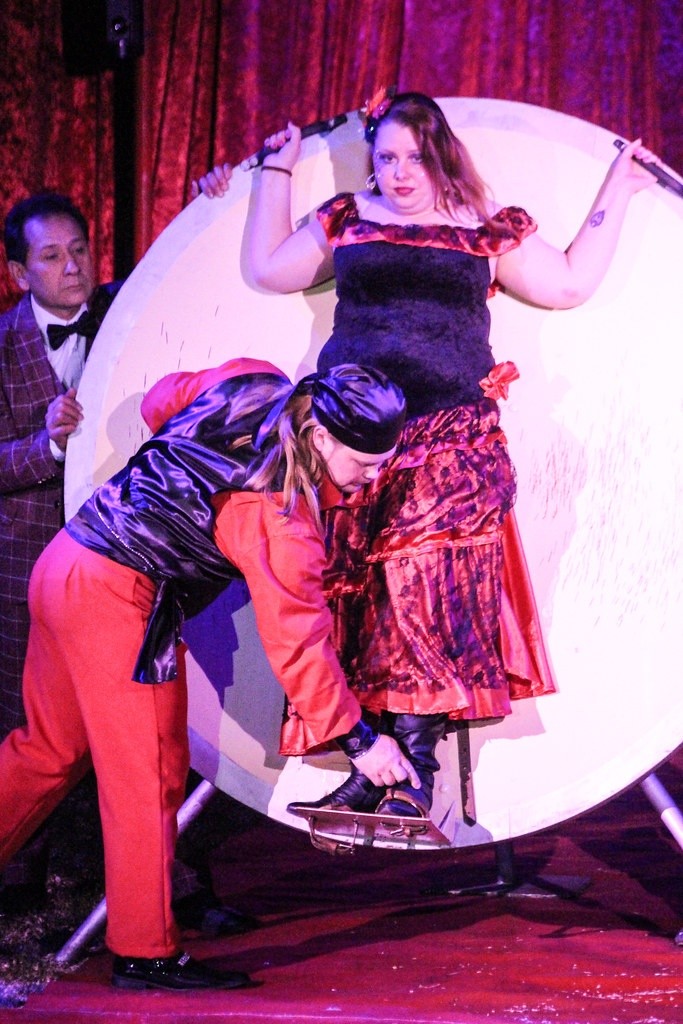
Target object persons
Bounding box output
[0,187,125,740]
[240,93,667,823]
[0,357,424,991]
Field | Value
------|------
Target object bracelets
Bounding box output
[259,163,292,177]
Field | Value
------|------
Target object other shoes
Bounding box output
[175,900,256,935]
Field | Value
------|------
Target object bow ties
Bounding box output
[46,310,99,351]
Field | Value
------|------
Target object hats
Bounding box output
[251,363,407,454]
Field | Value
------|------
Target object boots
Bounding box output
[386,714,448,816]
[285,760,386,818]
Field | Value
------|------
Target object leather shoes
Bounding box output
[111,951,250,990]
